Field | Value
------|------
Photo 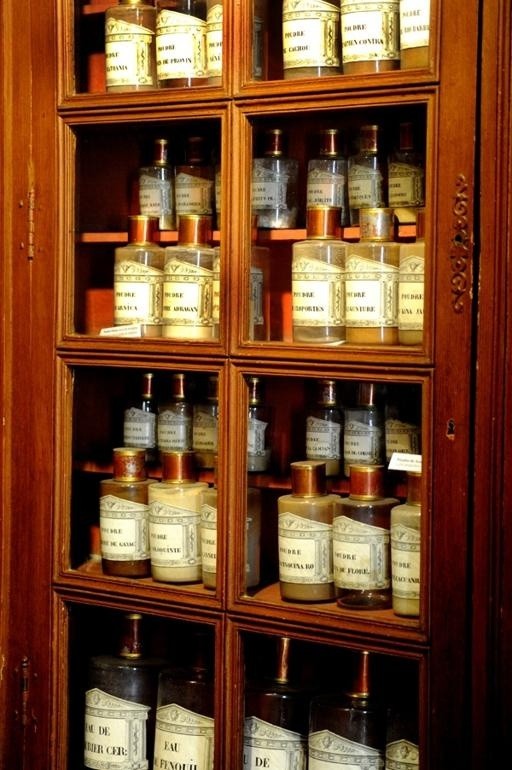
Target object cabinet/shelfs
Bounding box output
[0,0,512,770]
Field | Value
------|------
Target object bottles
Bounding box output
[136,140,177,232]
[199,454,262,590]
[390,471,420,618]
[154,622,218,769]
[344,208,404,347]
[343,382,384,484]
[277,459,343,605]
[255,129,299,230]
[281,1,343,81]
[246,376,272,476]
[400,2,432,70]
[123,373,159,473]
[383,384,421,483]
[113,214,164,340]
[98,446,160,579]
[104,2,158,94]
[175,136,214,232]
[241,636,313,770]
[340,2,400,75]
[205,1,223,88]
[333,464,403,612]
[307,129,350,229]
[82,614,163,770]
[212,245,272,348]
[346,124,390,229]
[161,212,213,343]
[303,379,344,484]
[193,377,220,475]
[308,651,386,770]
[292,205,350,347]
[156,373,193,472]
[385,711,420,770]
[398,208,427,347]
[146,447,208,585]
[212,168,222,232]
[154,0,207,88]
[387,122,427,223]
